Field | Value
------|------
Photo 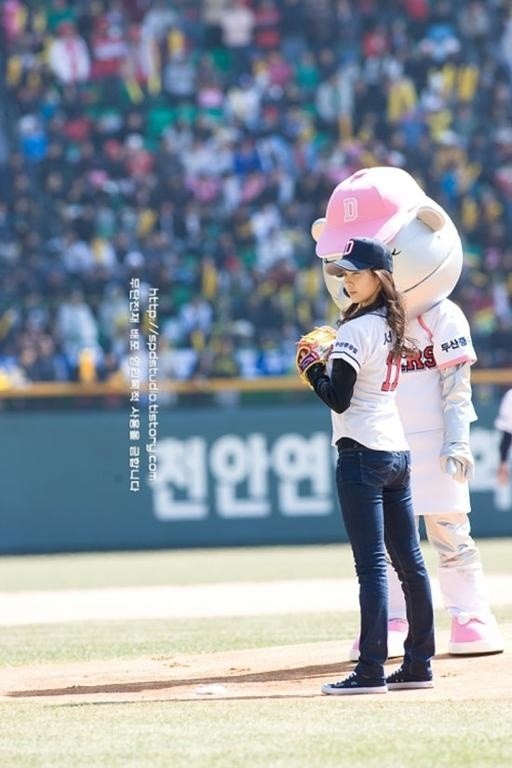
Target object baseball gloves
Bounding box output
[294,326,336,386]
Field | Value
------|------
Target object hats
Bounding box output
[325,238,393,274]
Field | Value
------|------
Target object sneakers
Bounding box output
[322,670,388,694]
[386,664,432,690]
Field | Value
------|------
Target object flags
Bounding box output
[311,165,506,656]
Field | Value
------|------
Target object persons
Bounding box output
[494,384,512,487]
[0,0,512,397]
[296,237,436,696]
